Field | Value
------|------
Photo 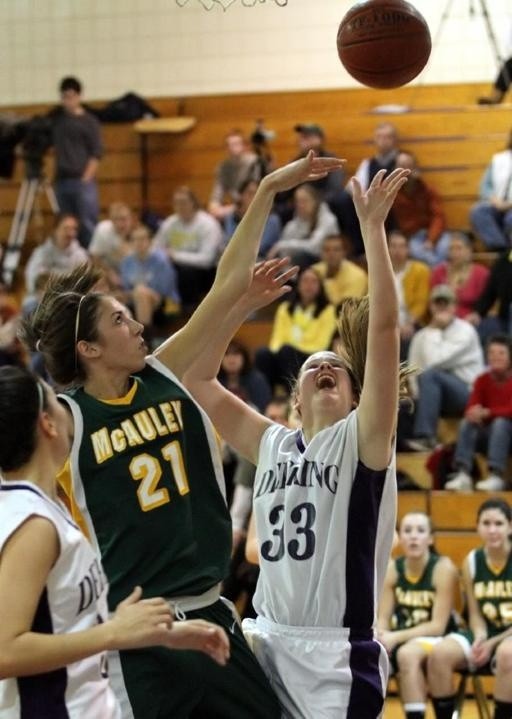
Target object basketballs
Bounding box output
[336,0,431,87]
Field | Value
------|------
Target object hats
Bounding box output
[290,123,326,138]
[427,283,457,300]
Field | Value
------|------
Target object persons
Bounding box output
[379,510,457,719]
[425,497,512,719]
[1,363,231,718]
[1,79,511,491]
[179,166,408,717]
[21,147,349,710]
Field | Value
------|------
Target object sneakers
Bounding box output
[475,474,506,491]
[443,470,474,491]
[400,435,439,455]
